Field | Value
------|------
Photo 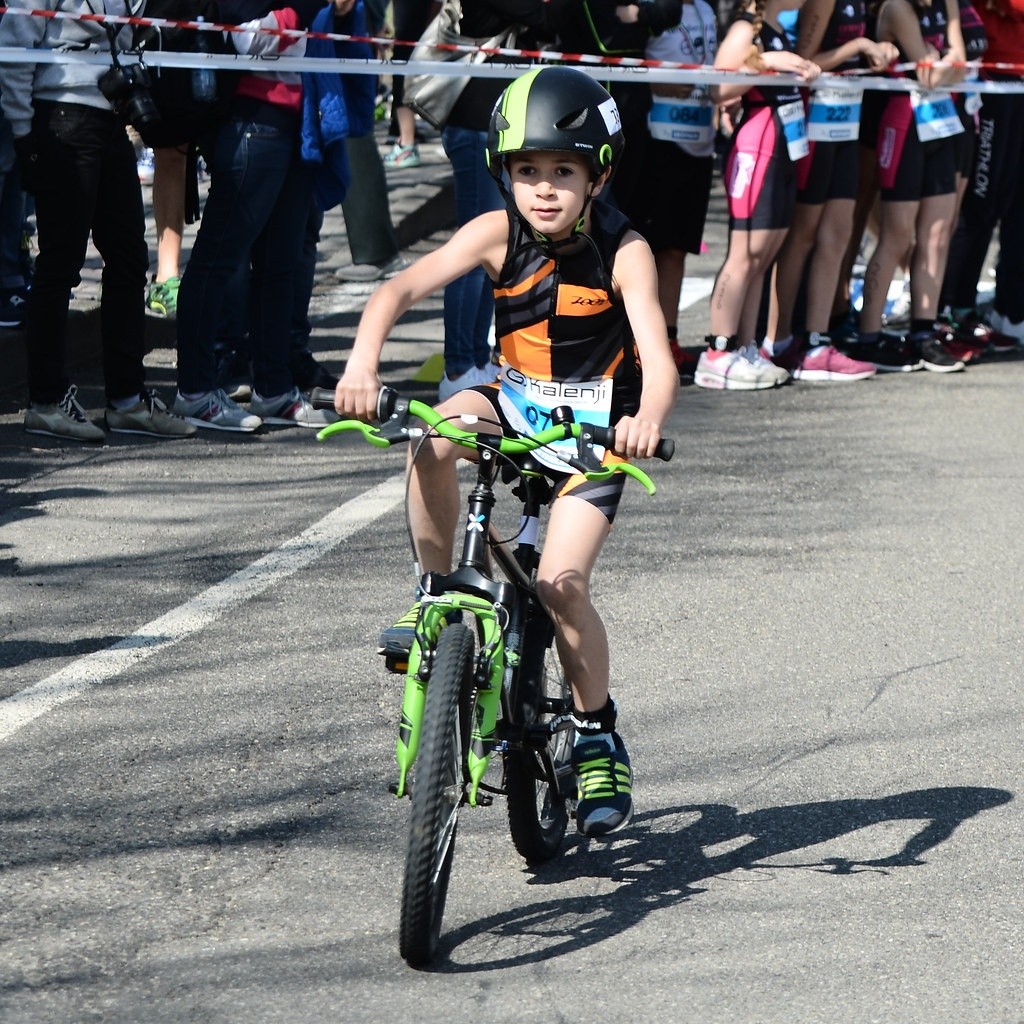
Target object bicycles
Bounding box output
[311,386,675,967]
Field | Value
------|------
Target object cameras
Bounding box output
[98,63,162,134]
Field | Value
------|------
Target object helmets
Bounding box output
[484,67,627,187]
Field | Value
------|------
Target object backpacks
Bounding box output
[100,0,232,154]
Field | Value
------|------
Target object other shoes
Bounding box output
[385,132,426,143]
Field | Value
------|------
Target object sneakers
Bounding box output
[666,338,695,385]
[435,352,504,402]
[568,729,632,839]
[757,307,1024,382]
[376,142,421,168]
[170,380,262,434]
[333,251,414,282]
[23,395,108,442]
[692,343,793,391]
[248,385,344,428]
[373,593,465,655]
[142,273,187,323]
[0,279,38,327]
[104,392,198,438]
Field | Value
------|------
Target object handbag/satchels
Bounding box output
[398,4,525,131]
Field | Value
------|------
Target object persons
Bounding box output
[334,67,679,840]
[693,0,1024,390]
[0,0,720,442]
